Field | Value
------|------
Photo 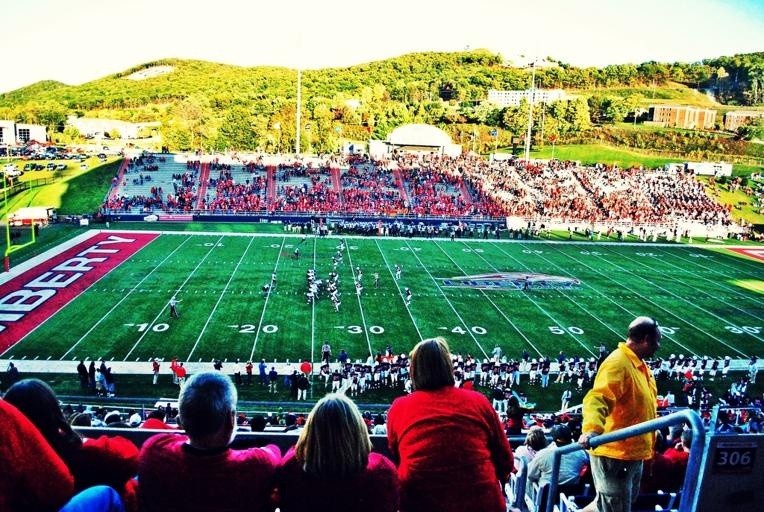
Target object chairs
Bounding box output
[504,458,598,512]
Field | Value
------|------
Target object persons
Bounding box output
[293,240,378,312]
[34,144,764,245]
[395,265,402,280]
[405,287,411,307]
[259,284,271,294]
[169,297,183,318]
[272,272,276,284]
[523,276,530,290]
[0,316,764,512]
